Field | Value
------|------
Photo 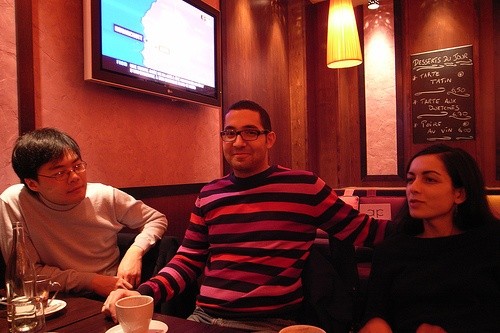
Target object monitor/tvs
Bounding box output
[82,0,222,110]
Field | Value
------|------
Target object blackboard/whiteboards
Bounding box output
[409,45,476,145]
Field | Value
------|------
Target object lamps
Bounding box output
[368,0,380,9]
[326,0,363,69]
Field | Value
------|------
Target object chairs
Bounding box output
[115,232,196,320]
[302,243,363,333]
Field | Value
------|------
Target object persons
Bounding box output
[102,101,390,333]
[0,127,179,304]
[358,143,500,333]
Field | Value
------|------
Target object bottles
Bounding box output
[5,221,37,323]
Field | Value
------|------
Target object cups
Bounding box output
[115,295,153,333]
[10,297,47,333]
[22,274,61,312]
[279,324,326,333]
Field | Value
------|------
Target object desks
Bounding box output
[52,306,254,333]
[0,283,105,333]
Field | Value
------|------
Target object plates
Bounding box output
[8,299,67,318]
[105,320,168,333]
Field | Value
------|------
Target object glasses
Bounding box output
[31,160,87,181]
[220,129,267,143]
